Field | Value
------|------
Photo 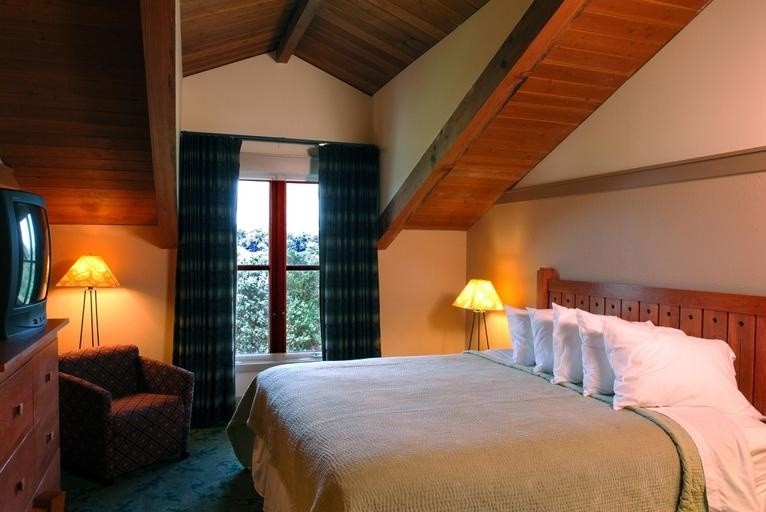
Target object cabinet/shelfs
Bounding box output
[0,318,69,512]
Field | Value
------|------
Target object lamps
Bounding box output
[56,256,121,349]
[451,279,505,352]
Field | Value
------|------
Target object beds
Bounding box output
[226,268,765,512]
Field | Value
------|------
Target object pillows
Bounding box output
[601,315,766,419]
[549,302,583,384]
[575,307,654,397]
[504,304,535,367]
[525,306,553,373]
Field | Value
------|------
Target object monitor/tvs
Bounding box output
[0,187,52,340]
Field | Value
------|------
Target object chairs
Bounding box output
[58,345,196,488]
